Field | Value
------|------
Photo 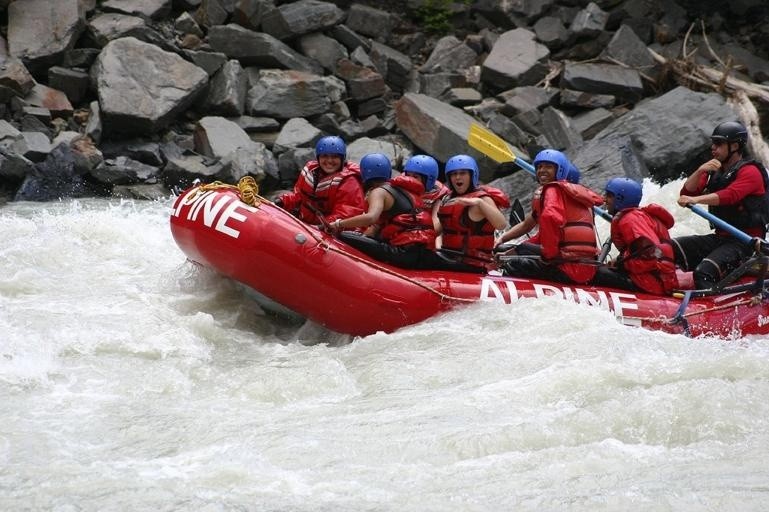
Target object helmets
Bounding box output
[403,155,439,192]
[566,161,580,184]
[360,153,392,183]
[445,155,479,189]
[316,136,346,159]
[710,122,748,139]
[533,149,569,181]
[605,177,642,216]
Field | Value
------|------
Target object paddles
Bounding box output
[467,122,613,222]
[509,199,532,239]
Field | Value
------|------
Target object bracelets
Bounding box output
[336,219,345,231]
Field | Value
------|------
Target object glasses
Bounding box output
[712,139,727,146]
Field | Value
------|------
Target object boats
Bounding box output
[169,176,769,337]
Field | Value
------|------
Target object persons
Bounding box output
[591,175,680,297]
[501,149,604,285]
[493,161,596,255]
[329,153,435,270]
[432,155,510,276]
[669,121,769,295]
[400,154,452,271]
[272,135,368,238]
